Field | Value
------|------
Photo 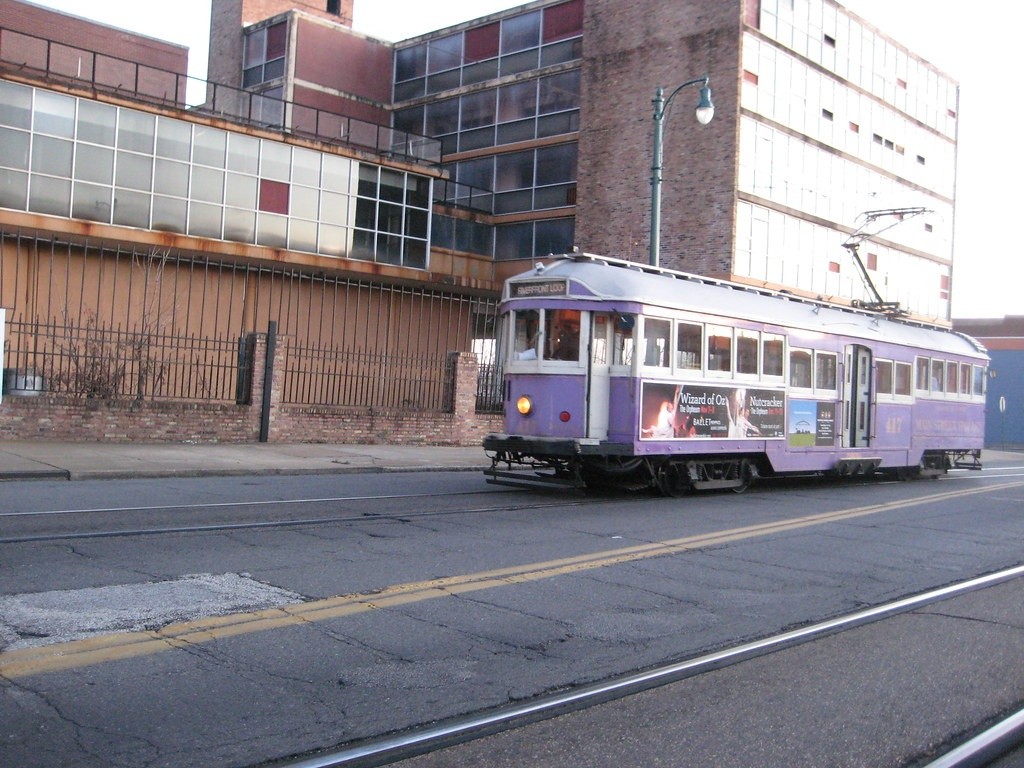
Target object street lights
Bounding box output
[650,79,715,268]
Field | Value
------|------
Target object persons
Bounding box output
[724,396,762,437]
[551,328,578,360]
[640,385,679,439]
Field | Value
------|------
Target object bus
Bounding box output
[483,249,992,492]
